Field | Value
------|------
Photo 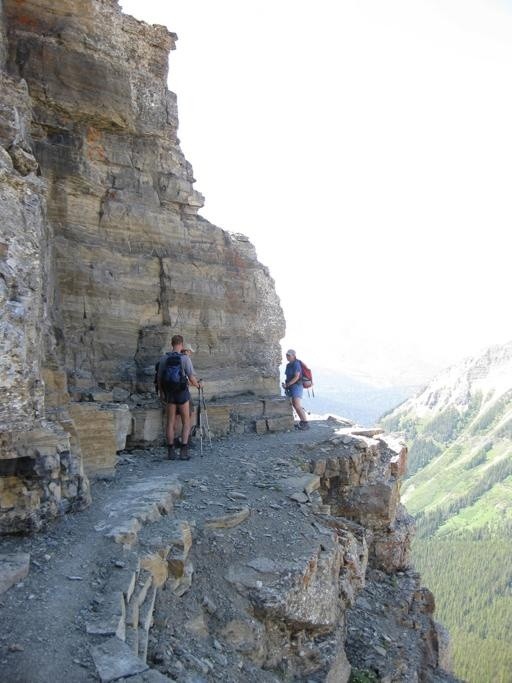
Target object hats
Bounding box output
[167,443,190,460]
[286,349,296,355]
[182,344,194,352]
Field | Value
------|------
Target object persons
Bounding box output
[281,349,309,430]
[169,343,197,448]
[157,334,204,460]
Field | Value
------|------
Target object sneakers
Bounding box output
[294,420,310,429]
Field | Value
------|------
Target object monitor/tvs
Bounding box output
[190,388,213,458]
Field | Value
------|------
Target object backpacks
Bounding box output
[160,352,185,400]
[287,360,313,389]
